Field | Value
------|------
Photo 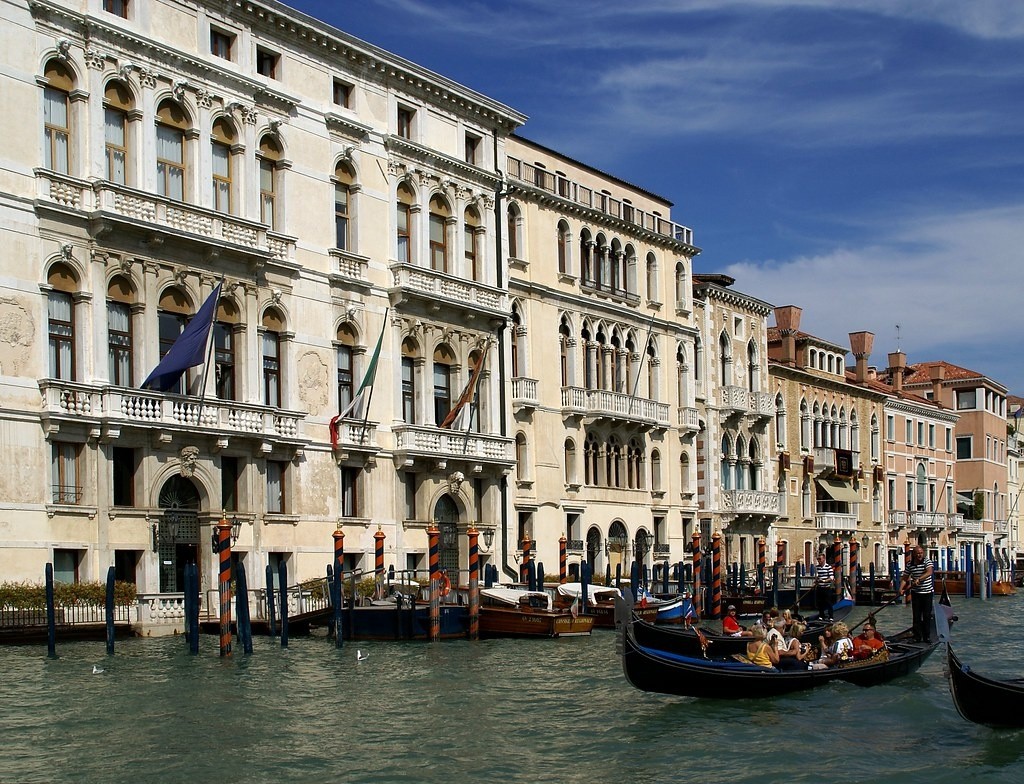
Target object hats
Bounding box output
[728,605,736,611]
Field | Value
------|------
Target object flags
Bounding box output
[329,326,384,451]
[440,348,484,429]
[139,283,221,392]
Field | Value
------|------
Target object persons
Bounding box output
[723,604,887,673]
[899,547,935,644]
[815,552,835,623]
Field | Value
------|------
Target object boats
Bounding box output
[610,575,959,698]
[501,579,662,628]
[608,578,699,625]
[933,597,1024,731]
[897,569,1018,597]
[412,581,600,638]
[622,576,856,641]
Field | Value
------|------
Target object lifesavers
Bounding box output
[436,574,451,597]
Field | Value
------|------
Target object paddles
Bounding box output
[785,578,823,611]
[847,580,921,634]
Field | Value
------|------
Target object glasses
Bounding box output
[817,558,821,560]
[863,629,874,632]
